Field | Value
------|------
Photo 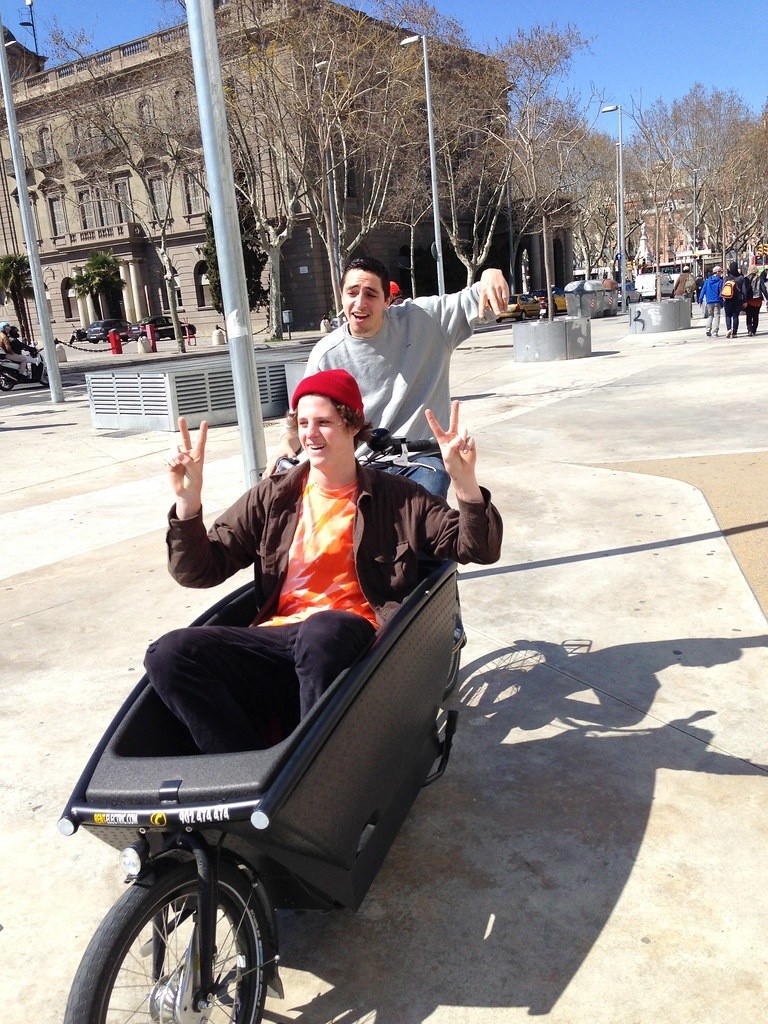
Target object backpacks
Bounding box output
[720,277,738,298]
[685,273,697,295]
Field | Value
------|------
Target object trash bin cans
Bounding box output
[564,280,606,320]
[596,279,619,316]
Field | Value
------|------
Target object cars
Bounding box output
[496,294,540,324]
[127,315,196,341]
[617,282,644,306]
[530,286,567,314]
[87,319,132,344]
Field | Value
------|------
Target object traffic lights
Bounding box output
[758,244,768,256]
[627,261,632,271]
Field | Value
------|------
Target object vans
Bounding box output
[634,272,675,301]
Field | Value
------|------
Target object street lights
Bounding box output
[693,169,700,252]
[601,105,626,314]
[315,60,341,285]
[615,142,625,255]
[400,35,446,297]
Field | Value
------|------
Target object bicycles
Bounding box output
[57,427,468,1024]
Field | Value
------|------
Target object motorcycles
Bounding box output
[69,324,87,344]
[0,341,50,391]
[538,291,556,319]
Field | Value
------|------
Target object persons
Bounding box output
[674,266,694,298]
[695,273,702,304]
[738,268,744,277]
[390,281,407,305]
[262,256,509,501]
[144,369,503,753]
[721,262,747,338]
[699,266,723,336]
[743,265,768,337]
[760,269,768,283]
[0,322,41,377]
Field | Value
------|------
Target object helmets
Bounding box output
[0,322,11,336]
[9,327,19,338]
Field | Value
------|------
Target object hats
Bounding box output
[683,266,690,272]
[747,265,759,274]
[293,370,364,421]
[713,266,723,273]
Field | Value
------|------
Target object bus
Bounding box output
[572,258,694,286]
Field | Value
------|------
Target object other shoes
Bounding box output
[749,331,754,336]
[714,333,718,337]
[706,330,712,337]
[726,330,732,339]
[732,334,738,337]
